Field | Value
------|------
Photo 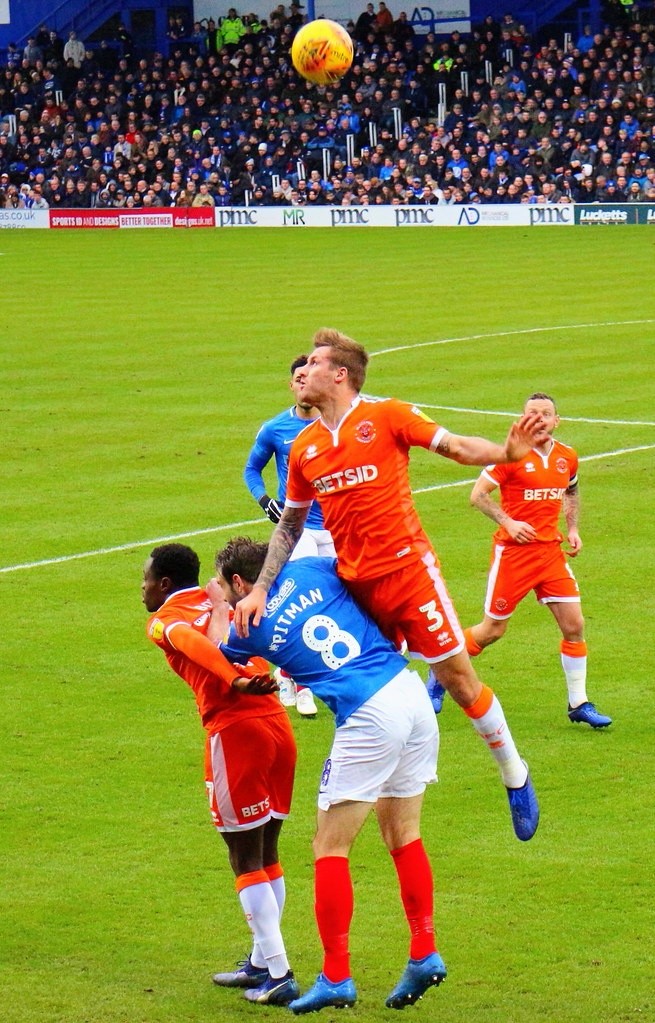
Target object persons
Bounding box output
[0,3,655,210]
[424,390,613,726]
[140,545,300,1003]
[244,354,338,714]
[210,536,452,1015]
[234,324,541,836]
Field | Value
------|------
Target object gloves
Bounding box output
[258,494,285,523]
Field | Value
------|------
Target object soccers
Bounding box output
[291,19,354,85]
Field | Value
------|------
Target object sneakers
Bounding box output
[245,967,300,1004]
[290,971,357,1015]
[272,667,296,707]
[292,677,318,715]
[212,953,271,986]
[425,666,445,713]
[504,758,541,841]
[385,950,447,1010]
[567,701,612,728]
